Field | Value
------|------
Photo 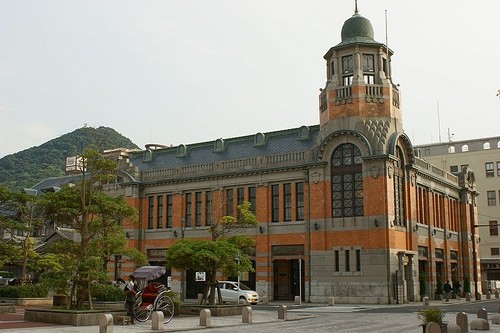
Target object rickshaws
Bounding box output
[128,264,176,324]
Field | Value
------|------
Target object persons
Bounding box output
[123,275,143,326]
[443,279,452,302]
[454,280,463,301]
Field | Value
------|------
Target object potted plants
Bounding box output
[418,308,449,333]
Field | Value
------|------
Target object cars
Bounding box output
[206,280,260,306]
[1,272,15,286]
